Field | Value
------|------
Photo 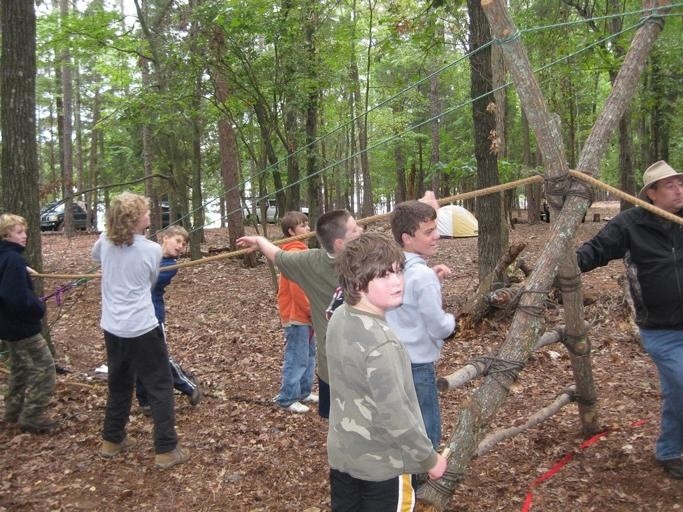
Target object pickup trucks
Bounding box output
[242,198,310,225]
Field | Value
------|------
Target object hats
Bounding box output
[637,160,683,199]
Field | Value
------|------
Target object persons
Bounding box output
[89,189,191,470]
[0,212,60,432]
[384,200,463,486]
[276,208,320,414]
[135,223,201,421]
[576,158,682,482]
[321,230,449,512]
[234,205,365,421]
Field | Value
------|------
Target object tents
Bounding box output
[430,204,478,241]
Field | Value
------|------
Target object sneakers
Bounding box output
[29,420,57,429]
[142,405,150,415]
[187,372,201,405]
[278,400,308,414]
[101,437,137,457]
[304,395,319,405]
[156,447,189,469]
[658,456,683,476]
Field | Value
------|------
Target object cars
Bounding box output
[149,201,191,231]
[39,202,97,231]
